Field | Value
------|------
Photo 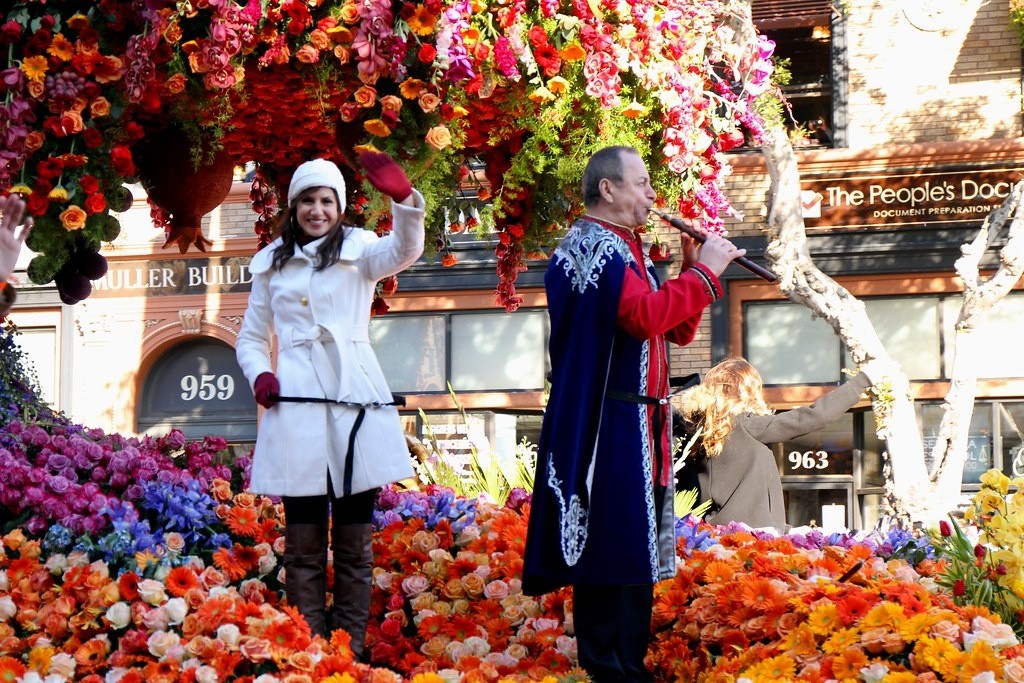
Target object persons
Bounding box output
[521,146,746,683]
[236,151,425,660]
[0,195,34,325]
[677,357,899,537]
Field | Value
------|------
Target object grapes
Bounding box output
[45,71,85,106]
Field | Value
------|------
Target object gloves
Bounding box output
[254,372,280,408]
[358,150,413,203]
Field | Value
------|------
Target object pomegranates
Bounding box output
[136,126,234,254]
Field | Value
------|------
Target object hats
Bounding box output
[288,158,345,214]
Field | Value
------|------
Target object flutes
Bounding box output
[647,208,779,284]
